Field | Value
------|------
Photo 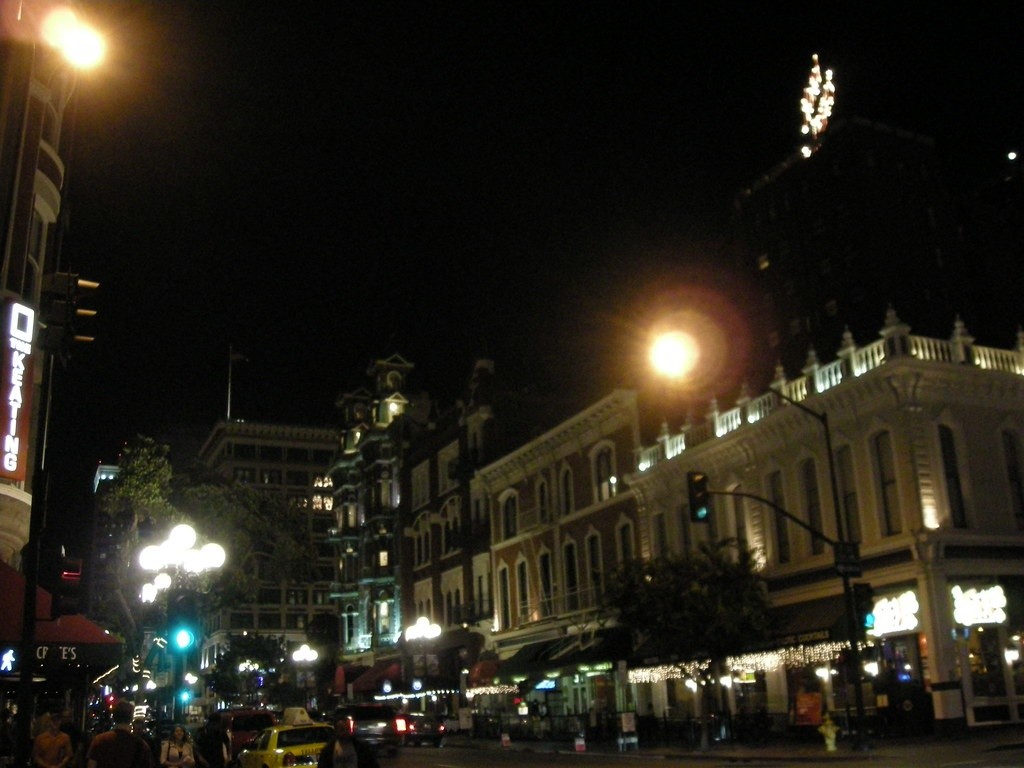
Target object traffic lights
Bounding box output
[1,646,21,673]
[181,686,190,705]
[37,271,101,352]
[167,586,200,656]
[688,468,711,524]
[854,583,875,630]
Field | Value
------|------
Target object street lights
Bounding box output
[650,333,866,749]
[292,644,318,709]
[406,616,442,714]
[139,525,226,724]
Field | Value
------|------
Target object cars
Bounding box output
[130,705,179,739]
[196,693,445,768]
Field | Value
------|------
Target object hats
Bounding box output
[50,713,63,724]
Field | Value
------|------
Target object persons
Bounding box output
[160,724,196,768]
[32,712,74,768]
[317,716,386,768]
[86,701,155,768]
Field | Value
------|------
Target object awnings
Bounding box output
[489,589,875,686]
